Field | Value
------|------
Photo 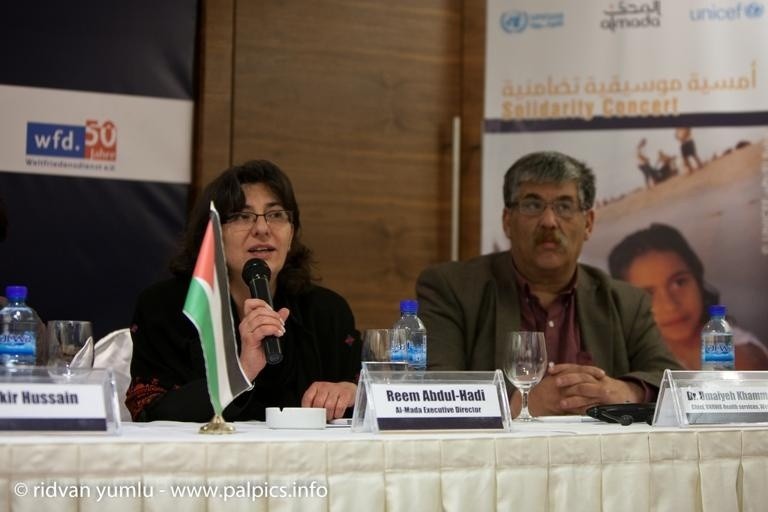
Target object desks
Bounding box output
[0,416,766,512]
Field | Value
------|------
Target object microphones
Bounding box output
[242,257,283,365]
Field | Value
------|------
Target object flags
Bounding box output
[181,198,256,420]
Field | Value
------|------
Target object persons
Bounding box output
[604,220,767,372]
[634,125,703,191]
[123,157,382,427]
[412,149,694,423]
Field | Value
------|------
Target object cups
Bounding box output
[44,318,94,385]
[359,327,406,385]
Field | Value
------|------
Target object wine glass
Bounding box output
[503,330,547,426]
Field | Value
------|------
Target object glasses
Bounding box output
[508,197,586,218]
[219,210,295,232]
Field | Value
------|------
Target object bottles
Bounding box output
[700,306,737,373]
[0,286,37,387]
[394,300,427,383]
